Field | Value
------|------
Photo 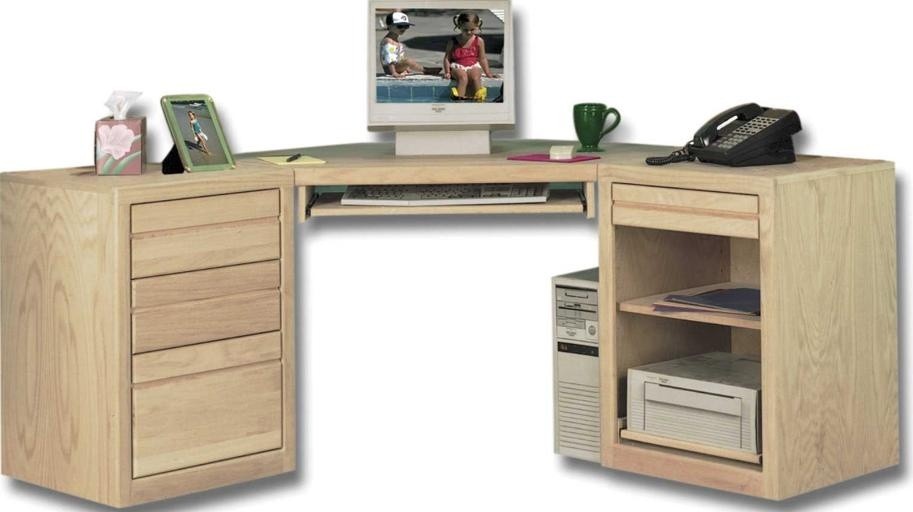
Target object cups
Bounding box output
[574,103,621,152]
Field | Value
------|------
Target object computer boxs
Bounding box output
[551,267,601,464]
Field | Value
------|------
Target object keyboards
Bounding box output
[340,185,551,206]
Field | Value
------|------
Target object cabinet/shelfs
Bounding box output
[0,143,899,511]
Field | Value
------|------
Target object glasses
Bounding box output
[397,24,409,29]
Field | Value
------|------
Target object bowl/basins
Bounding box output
[423,68,442,75]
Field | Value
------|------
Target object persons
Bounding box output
[379,11,442,78]
[188,110,211,155]
[443,10,500,99]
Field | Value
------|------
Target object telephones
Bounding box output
[686,103,802,167]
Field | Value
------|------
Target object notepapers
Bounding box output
[258,155,327,165]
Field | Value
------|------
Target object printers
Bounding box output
[627,351,762,454]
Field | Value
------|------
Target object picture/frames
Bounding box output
[161,94,236,172]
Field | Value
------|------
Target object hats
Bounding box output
[386,11,415,26]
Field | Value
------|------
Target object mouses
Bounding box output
[549,145,577,160]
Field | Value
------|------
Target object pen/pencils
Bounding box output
[286,153,301,162]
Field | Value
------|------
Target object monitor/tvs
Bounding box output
[368,0,516,157]
